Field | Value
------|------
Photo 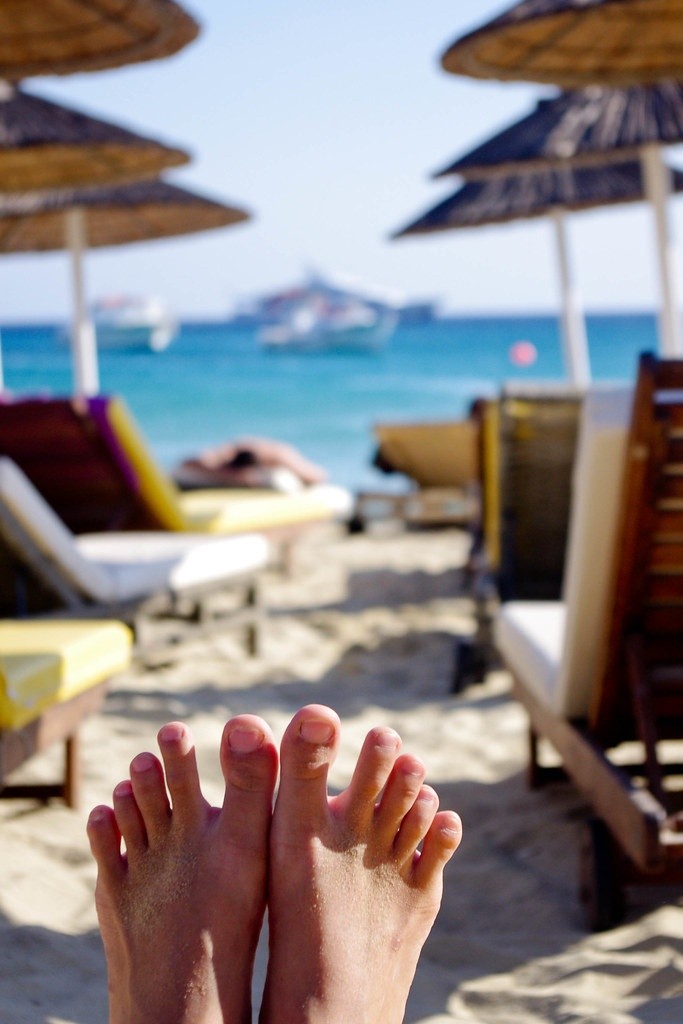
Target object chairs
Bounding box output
[461,394,583,675]
[0,616,133,806]
[2,389,354,612]
[375,421,473,525]
[175,460,303,493]
[0,458,269,670]
[490,350,682,933]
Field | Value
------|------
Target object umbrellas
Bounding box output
[391,0,683,393]
[0,0,252,394]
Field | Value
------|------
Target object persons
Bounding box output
[184,439,325,484]
[86,703,462,1024]
